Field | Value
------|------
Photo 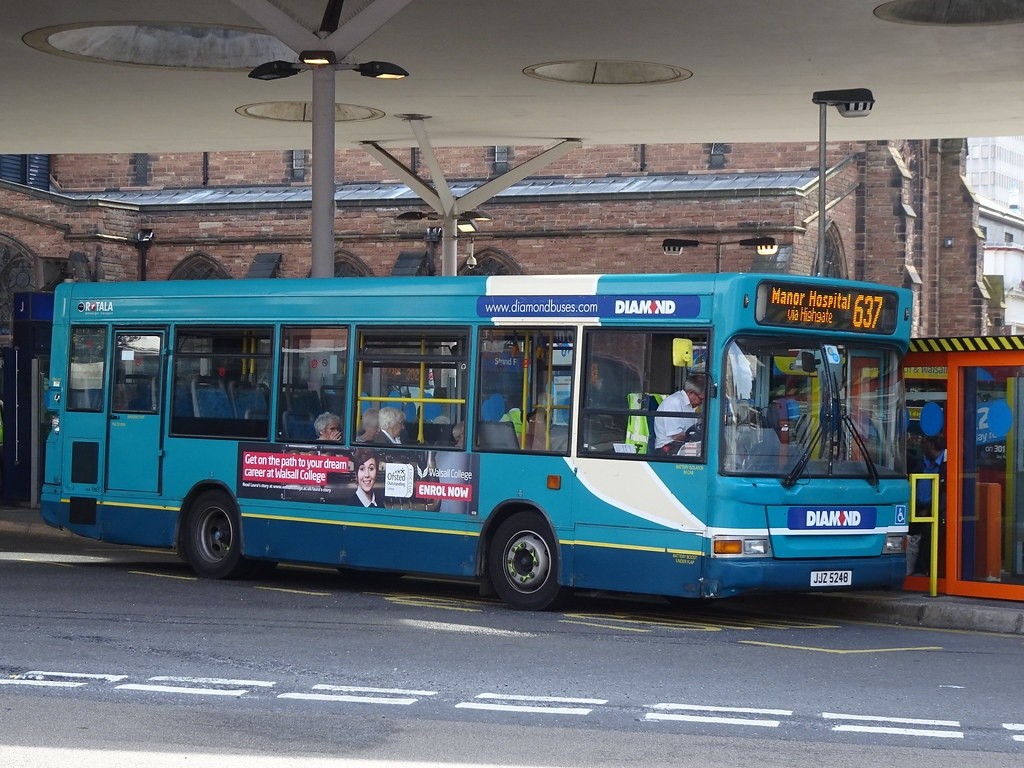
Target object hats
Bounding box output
[526,408,553,426]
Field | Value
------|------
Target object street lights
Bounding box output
[246,48,410,277]
[813,86,876,276]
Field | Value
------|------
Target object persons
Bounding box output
[498,395,529,439]
[654,375,706,453]
[356,392,465,446]
[906,431,948,576]
[346,448,386,508]
[314,414,342,441]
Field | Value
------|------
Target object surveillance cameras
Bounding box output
[466,256,477,269]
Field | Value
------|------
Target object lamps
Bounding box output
[944,237,954,248]
[248,49,408,81]
[399,207,492,233]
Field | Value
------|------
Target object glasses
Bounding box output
[697,394,704,402]
[529,420,543,424]
[325,427,341,431]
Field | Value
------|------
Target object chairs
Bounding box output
[70,371,670,455]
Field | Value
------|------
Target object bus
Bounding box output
[38,266,916,615]
